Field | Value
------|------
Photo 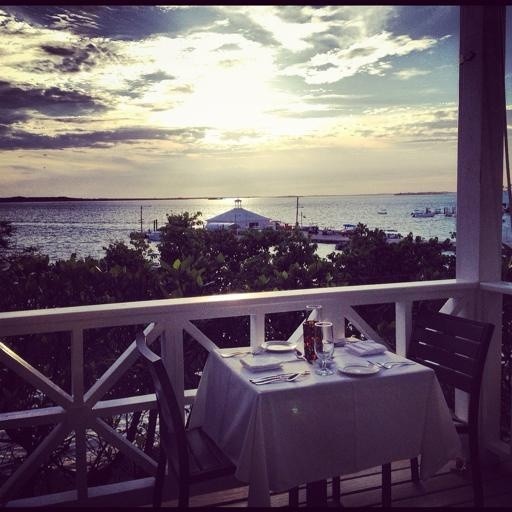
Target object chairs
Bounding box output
[136,330,299,512]
[381,301,495,512]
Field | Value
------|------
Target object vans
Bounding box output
[383,231,404,245]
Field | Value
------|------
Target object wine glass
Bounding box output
[313,322,335,375]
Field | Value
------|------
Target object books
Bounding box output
[334,337,361,347]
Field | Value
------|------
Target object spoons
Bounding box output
[255,373,301,385]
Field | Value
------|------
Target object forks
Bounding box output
[376,362,416,369]
[366,360,413,368]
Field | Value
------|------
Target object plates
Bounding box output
[338,362,380,375]
[261,341,297,352]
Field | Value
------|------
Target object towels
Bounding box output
[344,339,386,357]
[240,356,283,373]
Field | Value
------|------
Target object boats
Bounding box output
[409,205,455,218]
[377,208,387,214]
[207,198,224,200]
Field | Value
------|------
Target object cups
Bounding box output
[304,305,322,360]
